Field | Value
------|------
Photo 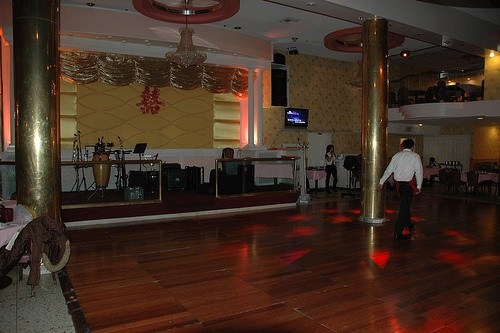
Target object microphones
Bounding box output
[332,151,336,157]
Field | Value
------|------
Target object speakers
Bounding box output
[343,156,359,169]
[262,62,289,109]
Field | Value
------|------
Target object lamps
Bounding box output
[354,43,362,87]
[166,0,206,69]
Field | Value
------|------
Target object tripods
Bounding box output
[341,169,359,198]
[71,130,100,200]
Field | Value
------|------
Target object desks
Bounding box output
[460,171,500,194]
[387,167,439,188]
[253,163,293,187]
[296,170,326,190]
[0,198,33,249]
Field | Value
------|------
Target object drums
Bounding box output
[92,151,110,189]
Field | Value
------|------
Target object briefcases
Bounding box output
[0,204,13,222]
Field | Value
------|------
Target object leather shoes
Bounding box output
[409,222,414,232]
[394,234,408,240]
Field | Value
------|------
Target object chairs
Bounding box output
[16,214,57,298]
[439,161,500,196]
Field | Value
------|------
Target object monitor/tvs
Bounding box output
[285,108,309,128]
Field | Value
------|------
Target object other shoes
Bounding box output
[327,189,338,193]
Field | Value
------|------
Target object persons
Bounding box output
[377,138,424,243]
[324,145,340,195]
